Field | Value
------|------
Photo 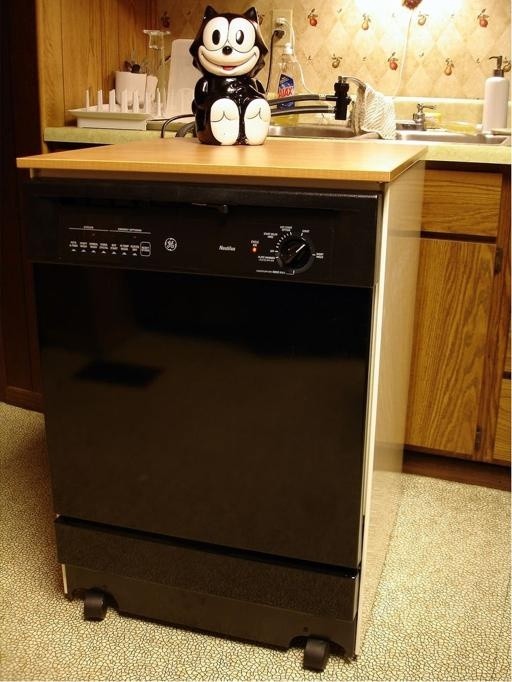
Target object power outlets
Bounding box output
[272,8,293,46]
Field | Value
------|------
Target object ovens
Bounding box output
[19,177,383,574]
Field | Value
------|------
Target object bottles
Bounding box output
[276,42,298,111]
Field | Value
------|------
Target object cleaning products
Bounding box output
[272,43,300,126]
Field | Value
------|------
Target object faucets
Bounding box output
[413,103,436,132]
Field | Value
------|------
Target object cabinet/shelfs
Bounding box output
[405,144,511,471]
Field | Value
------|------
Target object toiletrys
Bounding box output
[483,54,508,132]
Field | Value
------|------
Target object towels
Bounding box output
[346,84,397,139]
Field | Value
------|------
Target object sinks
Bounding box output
[266,120,372,140]
[353,126,509,148]
